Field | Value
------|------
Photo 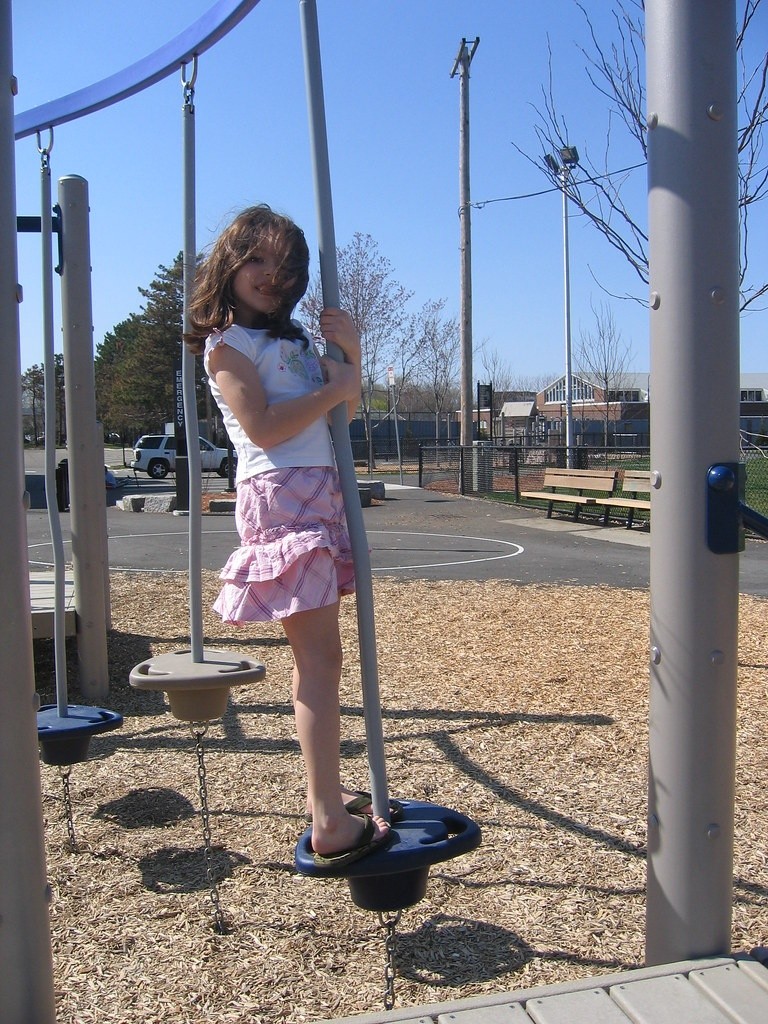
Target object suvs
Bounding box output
[129,432,239,481]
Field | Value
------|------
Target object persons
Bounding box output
[186,204,404,867]
[508,440,514,452]
[585,441,590,455]
[104,466,115,489]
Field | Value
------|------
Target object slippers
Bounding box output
[313,813,392,870]
[304,790,403,822]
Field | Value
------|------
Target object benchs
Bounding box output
[520,467,651,529]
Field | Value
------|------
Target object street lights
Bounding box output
[540,144,581,467]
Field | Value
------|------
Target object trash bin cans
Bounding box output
[59,459,70,508]
[472,440,494,493]
